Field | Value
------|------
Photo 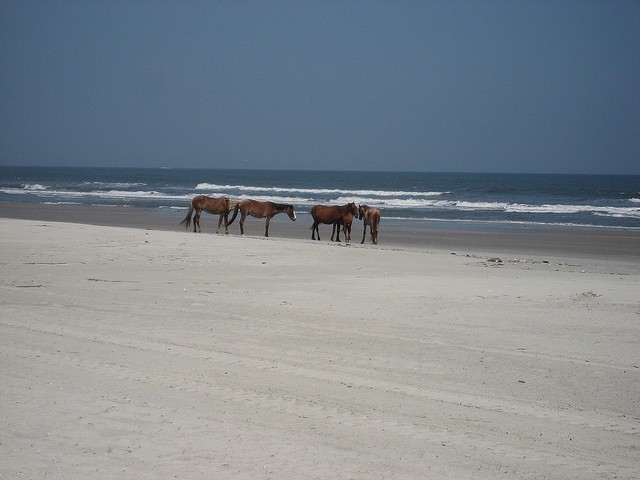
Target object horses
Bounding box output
[309,201,358,243]
[331,209,353,243]
[223,198,297,237]
[359,204,380,244]
[179,195,234,235]
